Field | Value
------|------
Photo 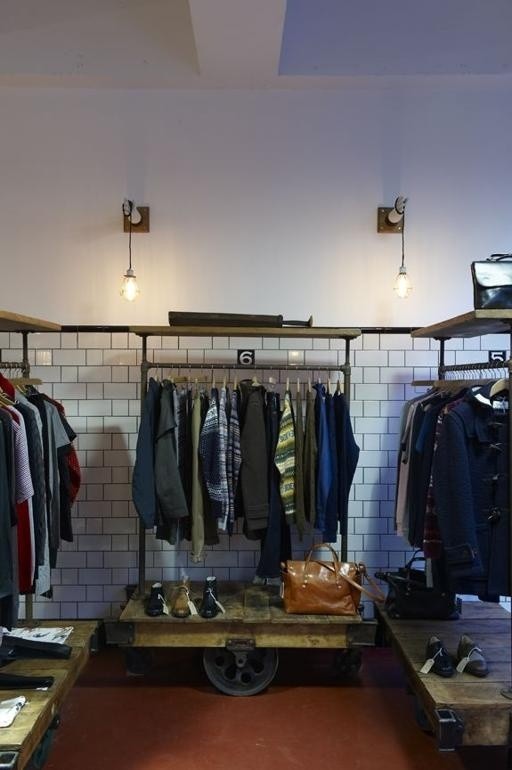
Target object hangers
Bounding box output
[421,365,510,389]
[0,358,43,392]
[147,361,352,395]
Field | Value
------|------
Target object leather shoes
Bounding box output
[425,634,454,679]
[457,633,490,679]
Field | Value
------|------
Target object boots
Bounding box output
[146,582,166,617]
[173,575,195,618]
[201,576,219,618]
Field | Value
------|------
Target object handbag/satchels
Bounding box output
[375,548,462,621]
[469,251,512,309]
[278,542,385,615]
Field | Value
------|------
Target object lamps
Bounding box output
[375,197,412,294]
[115,200,148,296]
